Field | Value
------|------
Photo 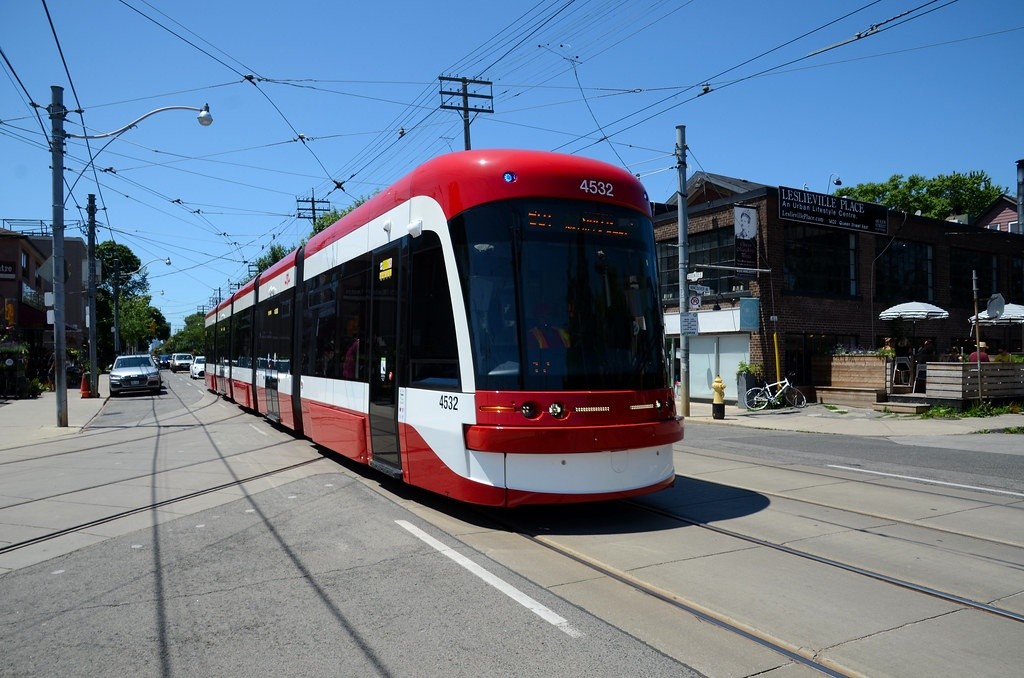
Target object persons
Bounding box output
[994,345,1010,362]
[916,339,937,378]
[967,342,991,362]
[946,347,963,362]
[734,209,756,239]
[525,302,573,363]
[897,333,913,361]
[878,338,896,360]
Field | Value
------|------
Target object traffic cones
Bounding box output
[79,373,91,399]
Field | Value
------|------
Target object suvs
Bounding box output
[169,353,195,373]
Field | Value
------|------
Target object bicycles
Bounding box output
[743,372,806,411]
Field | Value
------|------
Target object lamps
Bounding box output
[827,171,843,196]
[713,293,738,311]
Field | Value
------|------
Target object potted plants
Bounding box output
[18,377,43,399]
[736,359,762,411]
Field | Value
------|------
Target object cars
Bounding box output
[153,354,172,369]
[189,356,205,380]
[108,354,161,397]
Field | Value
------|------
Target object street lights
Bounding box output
[54,102,213,426]
[113,257,172,356]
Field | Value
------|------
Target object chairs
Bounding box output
[893,356,911,385]
[912,363,927,393]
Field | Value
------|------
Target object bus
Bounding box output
[202,147,685,507]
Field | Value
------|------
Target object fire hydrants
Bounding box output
[710,375,727,420]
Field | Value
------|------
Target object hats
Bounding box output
[974,341,989,349]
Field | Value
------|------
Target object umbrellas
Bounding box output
[968,303,1024,353]
[878,302,949,354]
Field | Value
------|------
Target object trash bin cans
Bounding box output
[84,372,100,398]
